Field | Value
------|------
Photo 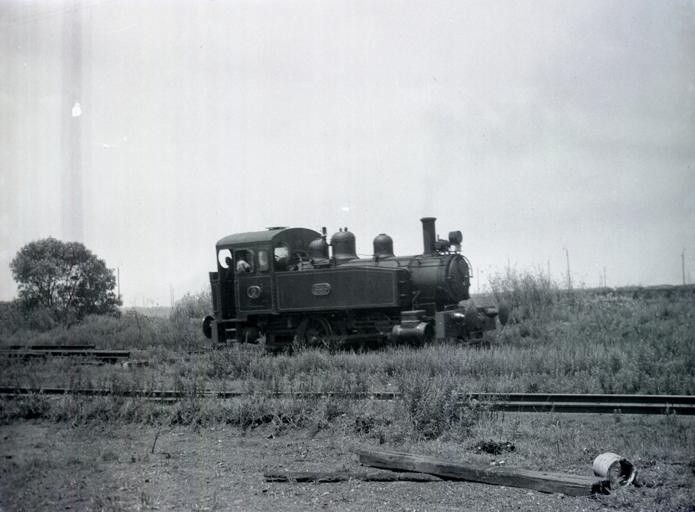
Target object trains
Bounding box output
[200,216,510,351]
